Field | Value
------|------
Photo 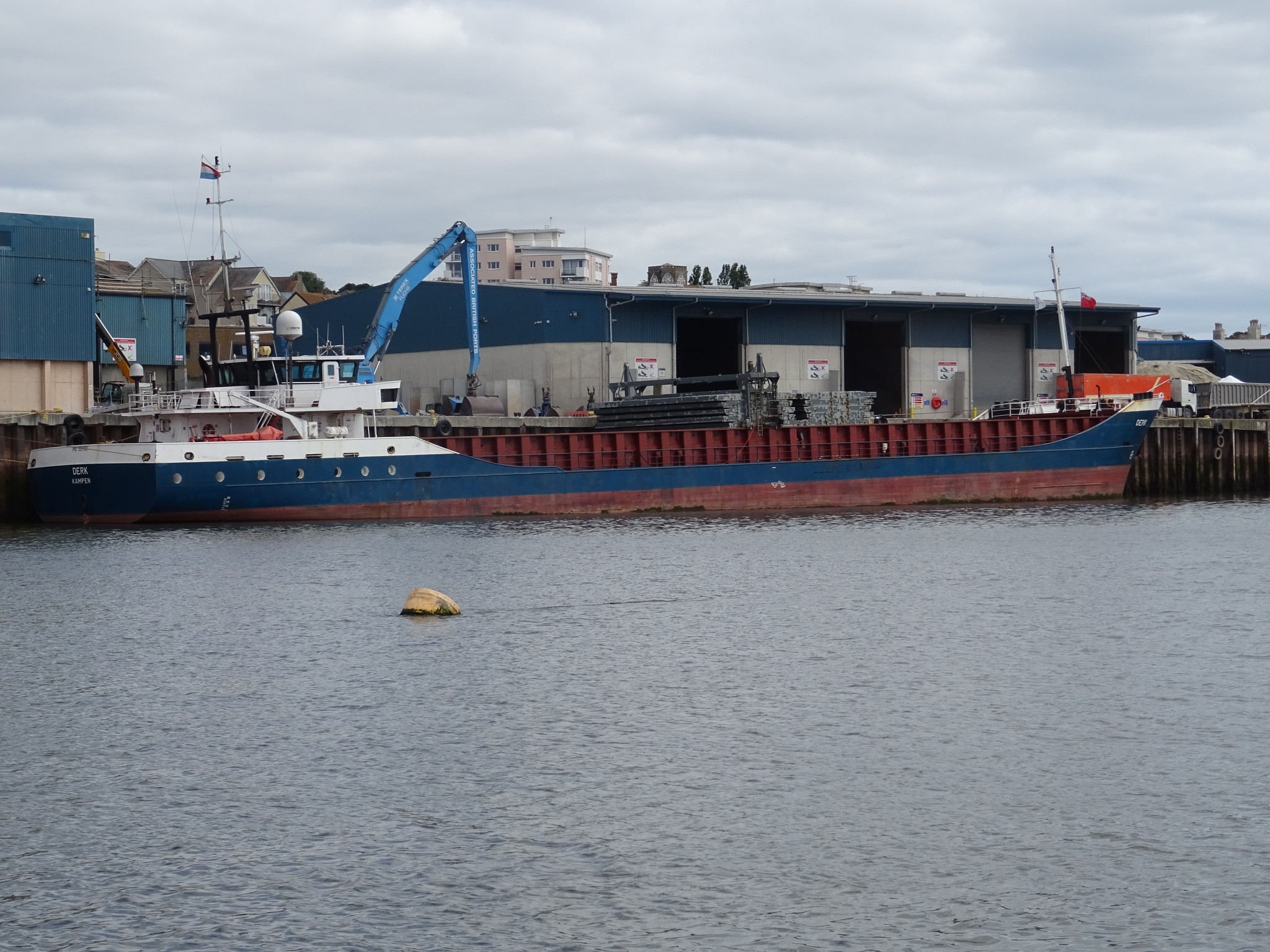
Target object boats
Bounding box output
[187,423,283,443]
[24,154,1169,528]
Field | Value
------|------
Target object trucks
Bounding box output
[1155,377,1198,418]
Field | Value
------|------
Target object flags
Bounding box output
[200,162,220,180]
[1081,292,1096,310]
[1035,296,1046,311]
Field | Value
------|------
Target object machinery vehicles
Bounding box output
[292,219,481,413]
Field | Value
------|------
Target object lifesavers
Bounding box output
[1212,447,1222,459]
[1214,423,1224,435]
[68,433,89,446]
[203,424,215,435]
[63,414,86,433]
[437,419,453,436]
[1215,435,1225,448]
